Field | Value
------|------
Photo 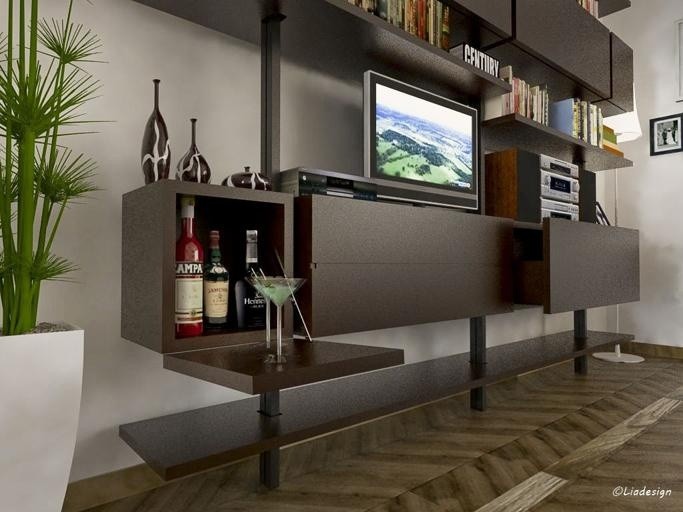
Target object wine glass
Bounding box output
[246,271,307,367]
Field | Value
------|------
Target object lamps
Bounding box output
[602,99,643,228]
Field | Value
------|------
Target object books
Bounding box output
[349,0,624,156]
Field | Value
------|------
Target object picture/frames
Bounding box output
[649,113,683,156]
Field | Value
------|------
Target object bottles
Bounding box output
[174,194,205,337]
[233,227,269,329]
[202,229,229,329]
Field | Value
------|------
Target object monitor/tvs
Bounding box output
[362,70,478,210]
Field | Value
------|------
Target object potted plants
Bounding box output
[0,0,111,512]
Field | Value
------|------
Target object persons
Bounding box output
[670,122,675,143]
[661,129,666,144]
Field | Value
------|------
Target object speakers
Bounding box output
[485,148,540,222]
[579,168,596,222]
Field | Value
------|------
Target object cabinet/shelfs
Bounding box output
[137,2,634,181]
[119,181,403,396]
[515,219,638,314]
[456,0,634,112]
[291,189,518,337]
